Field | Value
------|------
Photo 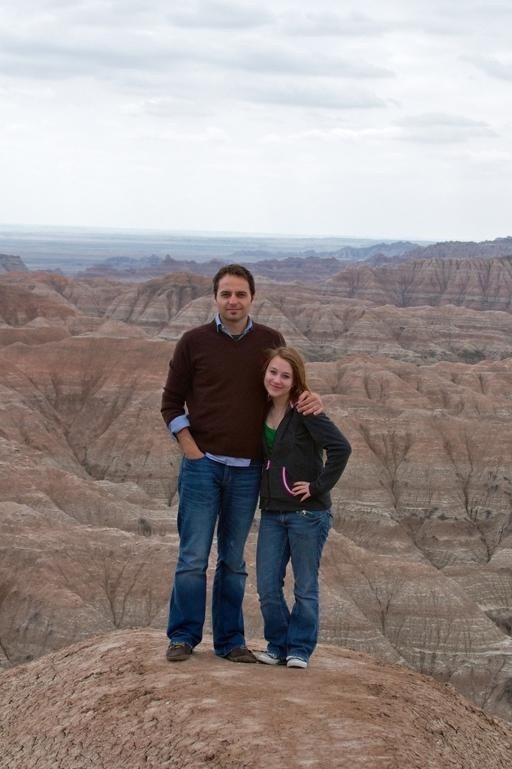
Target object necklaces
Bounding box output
[270,404,285,429]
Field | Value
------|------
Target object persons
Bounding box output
[251,344,352,669]
[161,263,325,664]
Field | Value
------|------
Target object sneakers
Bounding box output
[166,641,192,661]
[227,647,254,663]
[286,655,307,667]
[259,651,286,664]
